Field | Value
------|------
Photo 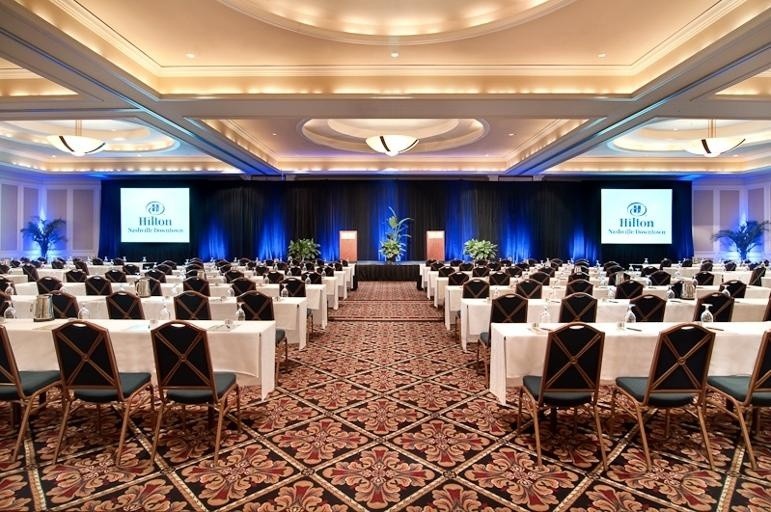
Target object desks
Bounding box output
[0,318,276,416]
[2,274,338,319]
[0,270,348,297]
[433,280,769,306]
[426,274,769,296]
[2,266,351,280]
[489,321,771,433]
[418,264,770,281]
[443,285,763,334]
[460,296,766,373]
[6,296,306,371]
[9,283,327,334]
[0,263,355,274]
[422,268,769,284]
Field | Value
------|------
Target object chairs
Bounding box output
[575,261,589,267]
[450,259,463,267]
[38,257,47,264]
[74,258,81,265]
[312,258,324,266]
[515,263,530,271]
[56,258,66,264]
[186,261,203,268]
[22,264,33,275]
[52,261,63,269]
[500,260,511,268]
[186,264,202,272]
[219,264,234,276]
[106,291,145,320]
[603,261,619,271]
[123,264,139,275]
[287,260,299,267]
[302,272,322,284]
[477,293,528,389]
[303,262,315,271]
[649,271,671,285]
[706,329,771,470]
[225,270,244,283]
[189,258,202,262]
[693,272,714,286]
[174,291,212,320]
[161,260,177,270]
[454,279,489,347]
[538,266,554,278]
[545,262,558,271]
[515,279,542,299]
[150,321,241,466]
[145,269,166,282]
[274,263,288,275]
[725,262,736,271]
[608,273,630,286]
[669,280,682,298]
[439,266,455,277]
[693,292,734,322]
[113,258,123,265]
[186,270,206,281]
[473,267,490,277]
[266,260,274,266]
[237,258,249,267]
[279,278,314,344]
[724,260,731,264]
[0,291,14,318]
[703,260,712,265]
[572,267,589,274]
[682,258,692,267]
[558,292,597,323]
[183,277,211,296]
[0,276,16,294]
[522,259,534,267]
[44,290,79,318]
[85,276,112,295]
[748,263,759,270]
[459,262,473,271]
[568,272,590,282]
[564,279,593,296]
[105,269,126,282]
[516,323,607,469]
[486,262,501,271]
[641,266,658,276]
[749,266,766,286]
[237,290,288,387]
[426,259,436,267]
[615,280,644,299]
[134,276,162,296]
[341,260,348,267]
[505,266,522,278]
[263,271,284,284]
[762,291,771,321]
[0,263,10,274]
[28,269,39,282]
[316,268,326,278]
[610,322,715,471]
[70,268,87,282]
[529,272,550,285]
[93,258,103,265]
[215,261,227,270]
[606,265,625,277]
[489,272,510,286]
[245,262,256,270]
[627,294,666,322]
[76,263,89,275]
[575,259,589,263]
[20,257,30,263]
[155,264,172,275]
[475,260,487,267]
[288,265,301,276]
[11,261,20,268]
[327,266,334,276]
[230,277,256,296]
[31,261,42,268]
[37,277,62,294]
[744,260,751,264]
[442,272,469,328]
[334,263,343,271]
[254,265,269,276]
[551,258,563,267]
[718,279,747,298]
[431,261,444,271]
[2,324,67,461]
[51,321,155,465]
[701,262,712,271]
[763,260,770,267]
[660,260,671,270]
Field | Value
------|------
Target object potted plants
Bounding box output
[377,207,416,265]
